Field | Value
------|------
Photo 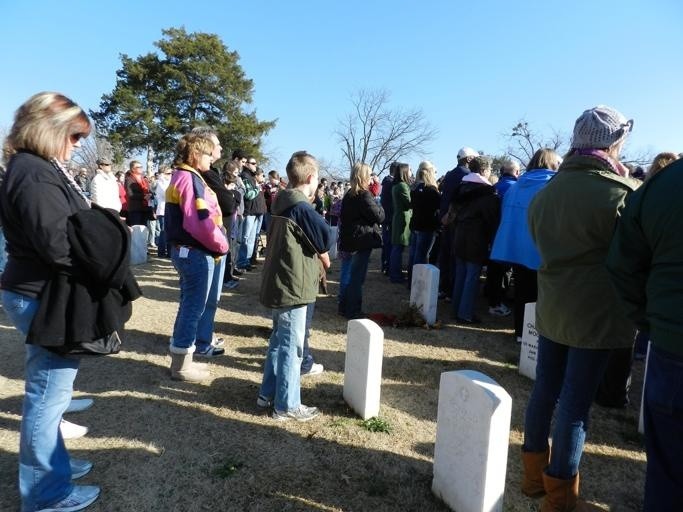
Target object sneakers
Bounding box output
[390,276,512,326]
[60,419,89,439]
[65,399,93,413]
[170,336,324,422]
[68,458,93,479]
[222,259,258,289]
[36,485,101,511]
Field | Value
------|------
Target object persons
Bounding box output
[0,92,143,510]
[1,91,384,439]
[615,159,682,511]
[522,106,645,512]
[380,146,682,406]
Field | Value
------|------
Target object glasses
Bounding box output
[69,133,81,143]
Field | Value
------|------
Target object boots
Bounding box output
[537,464,590,512]
[520,444,549,498]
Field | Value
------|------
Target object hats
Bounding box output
[458,146,479,160]
[569,105,634,148]
